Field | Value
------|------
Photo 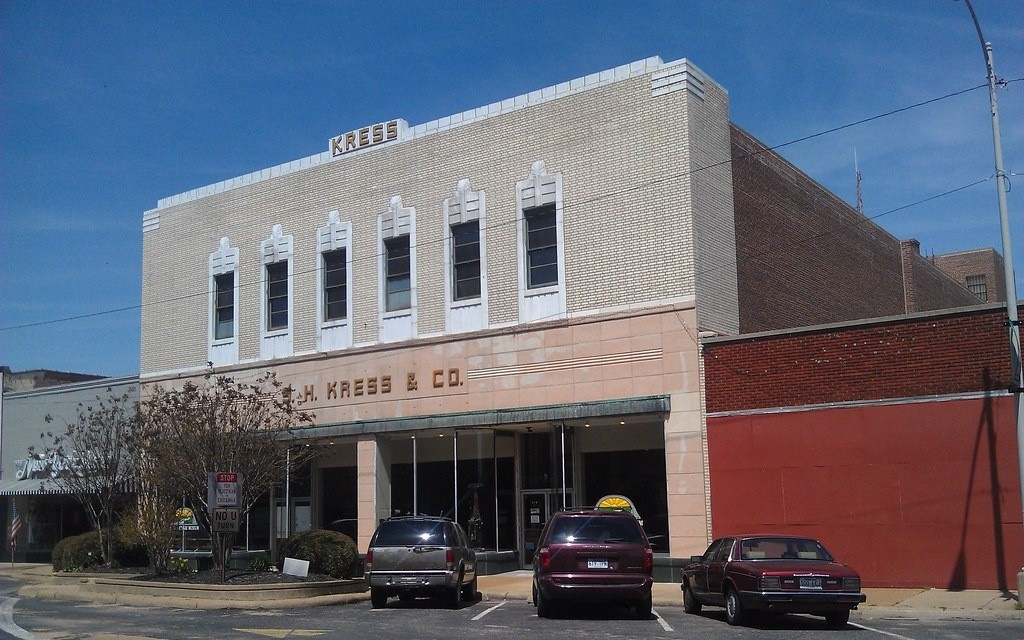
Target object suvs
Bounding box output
[363,516,478,610]
[531,507,655,618]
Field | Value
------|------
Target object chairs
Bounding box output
[746,551,766,559]
[798,551,817,559]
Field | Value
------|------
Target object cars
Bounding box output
[681,535,867,627]
